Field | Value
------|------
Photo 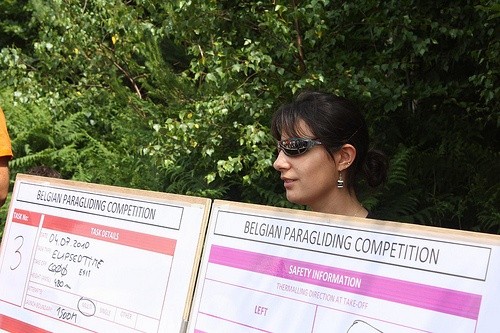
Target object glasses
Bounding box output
[276,138,338,157]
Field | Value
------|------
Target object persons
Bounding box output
[0,107,13,206]
[29,167,62,178]
[272,92,386,222]
